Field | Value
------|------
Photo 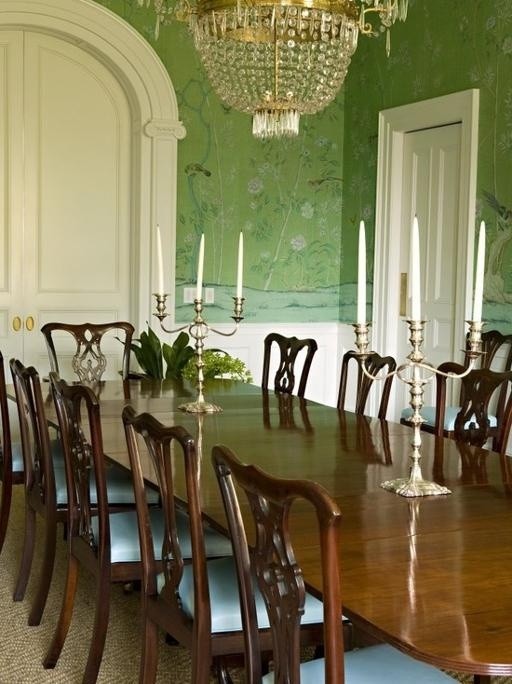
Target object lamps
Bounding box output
[138,1,408,141]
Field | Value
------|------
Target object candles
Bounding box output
[412,214,420,320]
[358,220,366,323]
[157,224,163,294]
[237,229,243,296]
[196,231,205,300]
[473,220,485,321]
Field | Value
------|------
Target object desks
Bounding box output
[6,374,509,680]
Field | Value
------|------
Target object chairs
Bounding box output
[119,401,356,684]
[209,442,473,684]
[334,348,398,420]
[39,370,239,683]
[258,332,317,399]
[39,320,138,380]
[430,360,508,458]
[0,349,56,552]
[5,355,161,622]
[398,326,511,444]
